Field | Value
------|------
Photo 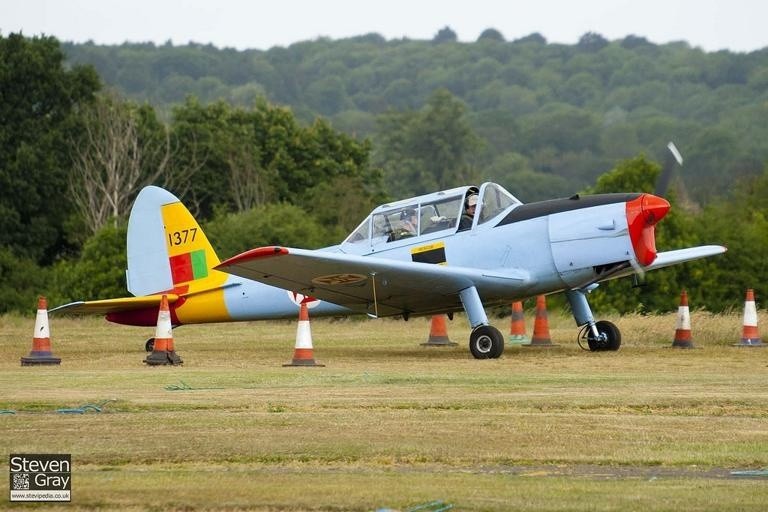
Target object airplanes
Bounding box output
[42,131,739,361]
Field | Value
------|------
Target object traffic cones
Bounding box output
[278,296,328,369]
[663,290,698,351]
[414,310,455,350]
[525,293,565,352]
[504,300,523,346]
[723,285,768,349]
[137,292,185,367]
[17,294,68,365]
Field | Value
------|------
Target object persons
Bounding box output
[386,209,420,243]
[456,192,485,232]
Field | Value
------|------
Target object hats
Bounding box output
[401,207,423,221]
[463,193,482,209]
